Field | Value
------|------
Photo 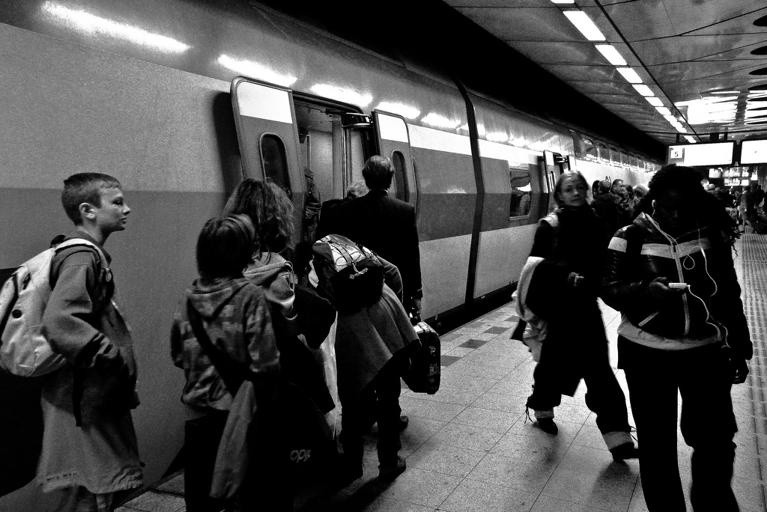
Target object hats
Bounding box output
[649,165,704,195]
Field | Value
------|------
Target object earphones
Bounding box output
[651,200,656,208]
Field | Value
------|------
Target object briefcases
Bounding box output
[402,312,443,397]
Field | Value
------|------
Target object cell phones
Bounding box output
[668,282,688,289]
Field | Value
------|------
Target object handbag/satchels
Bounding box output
[290,280,335,352]
[211,372,254,503]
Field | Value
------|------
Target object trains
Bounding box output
[0,0,668,512]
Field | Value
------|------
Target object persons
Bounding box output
[331,155,425,432]
[35,171,146,512]
[601,164,755,512]
[299,232,424,487]
[590,178,767,247]
[220,177,345,510]
[523,170,641,463]
[300,164,371,244]
[170,213,284,511]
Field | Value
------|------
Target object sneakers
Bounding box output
[380,414,408,435]
[378,452,406,483]
[536,413,560,437]
[613,443,640,461]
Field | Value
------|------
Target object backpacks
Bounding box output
[0,245,67,378]
[310,229,386,314]
